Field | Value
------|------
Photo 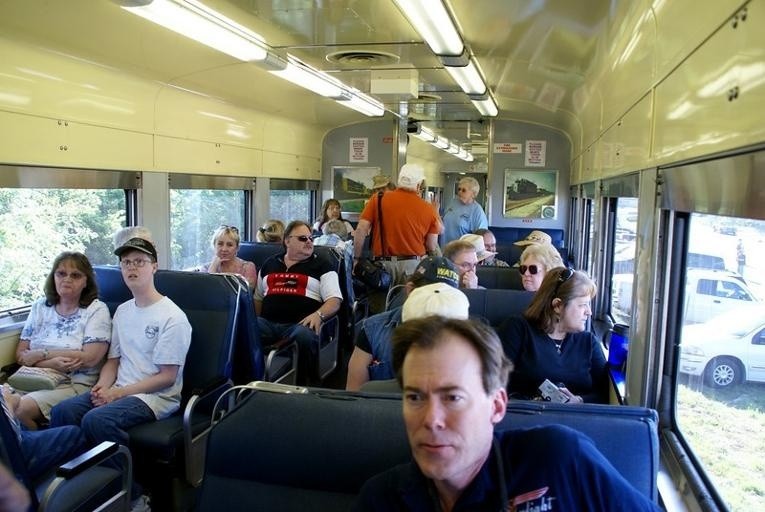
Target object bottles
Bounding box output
[603,323,630,369]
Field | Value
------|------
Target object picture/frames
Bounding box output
[502,168,560,220]
[331,165,381,215]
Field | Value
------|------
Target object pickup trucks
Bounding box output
[613,263,754,321]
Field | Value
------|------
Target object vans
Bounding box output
[613,240,727,271]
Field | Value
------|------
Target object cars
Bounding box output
[679,307,765,390]
[712,216,739,235]
[588,204,638,251]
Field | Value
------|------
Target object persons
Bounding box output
[210,198,357,387]
[735,238,745,277]
[349,163,440,314]
[416,179,445,235]
[438,175,488,255]
[1,229,193,512]
[345,229,663,512]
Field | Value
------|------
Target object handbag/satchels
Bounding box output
[354,257,391,290]
[7,365,72,391]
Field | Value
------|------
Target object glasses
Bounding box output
[222,225,239,234]
[119,259,152,266]
[555,268,574,296]
[288,235,314,242]
[519,265,538,274]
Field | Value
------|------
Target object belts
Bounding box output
[375,255,417,261]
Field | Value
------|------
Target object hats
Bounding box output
[401,256,470,323]
[398,163,426,184]
[512,230,552,245]
[114,238,157,262]
[460,233,498,261]
